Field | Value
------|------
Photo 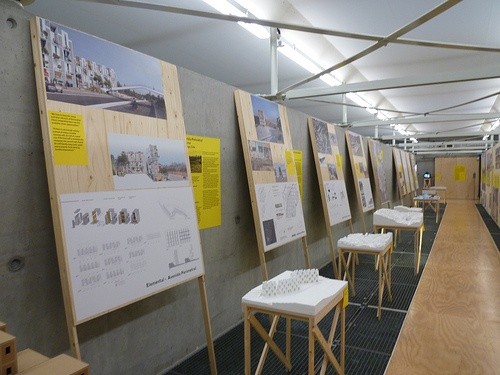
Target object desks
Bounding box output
[373,220,423,275]
[241,270,350,375]
[337,232,394,320]
[413,196,440,223]
[430,187,446,205]
